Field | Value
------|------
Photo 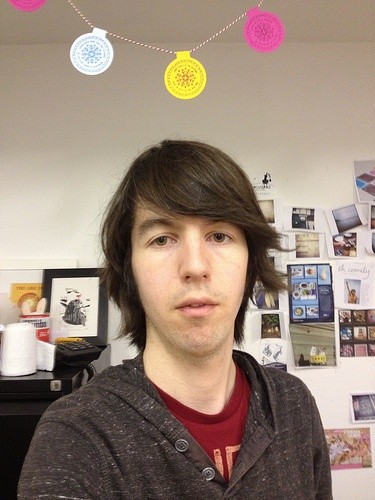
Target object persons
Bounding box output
[17,141,332,500]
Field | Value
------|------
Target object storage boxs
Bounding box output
[19,313,51,344]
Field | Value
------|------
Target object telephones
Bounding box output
[53,337,101,362]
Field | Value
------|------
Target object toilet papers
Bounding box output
[0,322,56,377]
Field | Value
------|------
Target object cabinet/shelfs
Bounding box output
[0,343,111,500]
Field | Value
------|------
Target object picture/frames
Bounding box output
[41,268,108,347]
